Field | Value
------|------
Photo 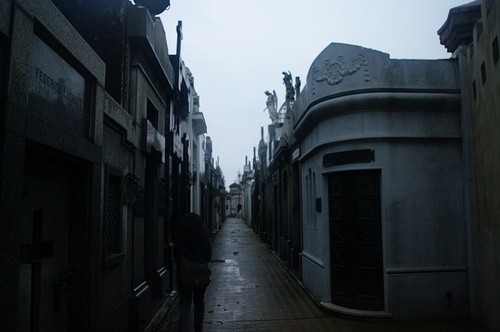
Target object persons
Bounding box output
[173,212,213,332]
[282,71,295,100]
[265,90,279,122]
